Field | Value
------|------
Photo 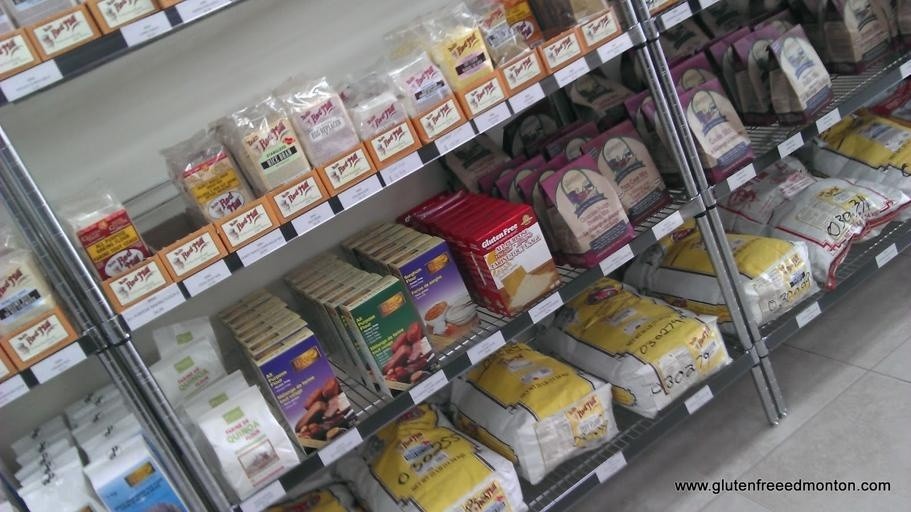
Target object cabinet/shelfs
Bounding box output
[0,0,911,510]
